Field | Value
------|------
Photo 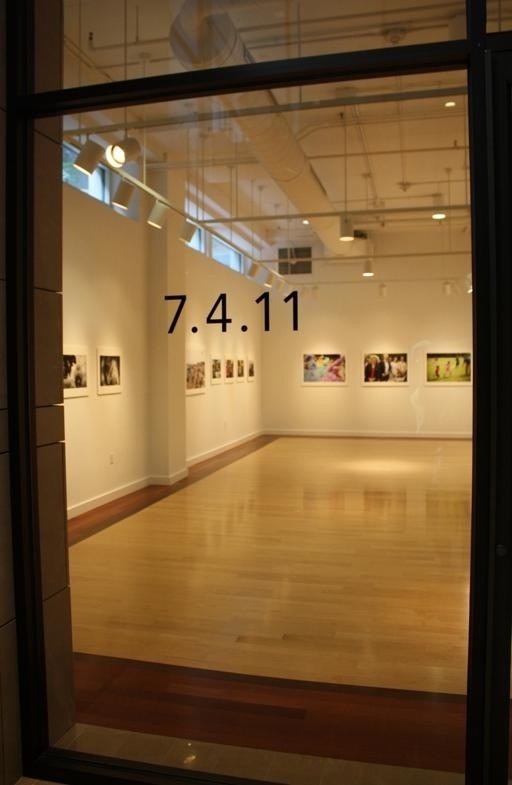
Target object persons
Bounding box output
[64,359,85,386]
[367,354,407,383]
[102,356,120,384]
[433,354,470,384]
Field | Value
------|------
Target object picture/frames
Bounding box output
[62,345,91,398]
[360,350,412,386]
[97,345,124,395]
[185,354,255,395]
[422,350,473,386]
[301,351,348,386]
